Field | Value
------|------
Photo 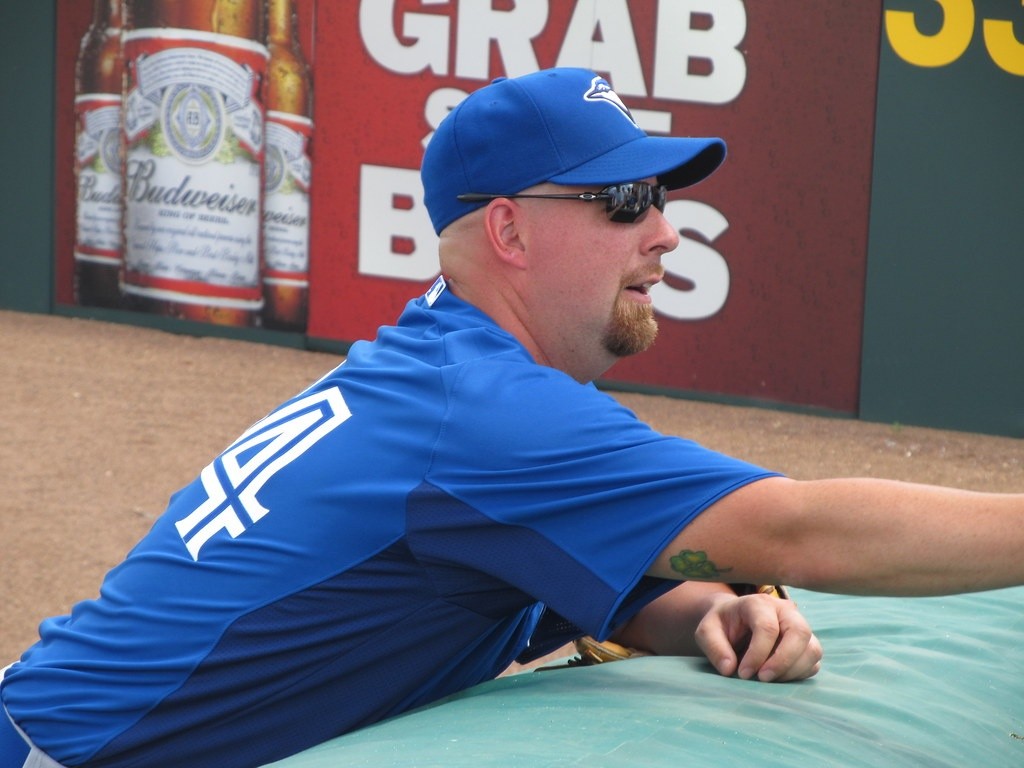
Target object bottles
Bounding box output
[120,1,266,335]
[253,0,313,333]
[72,0,141,311]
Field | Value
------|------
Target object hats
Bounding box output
[421,68,728,238]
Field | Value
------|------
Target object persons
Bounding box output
[0,67,1024,768]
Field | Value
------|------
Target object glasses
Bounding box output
[457,182,666,224]
